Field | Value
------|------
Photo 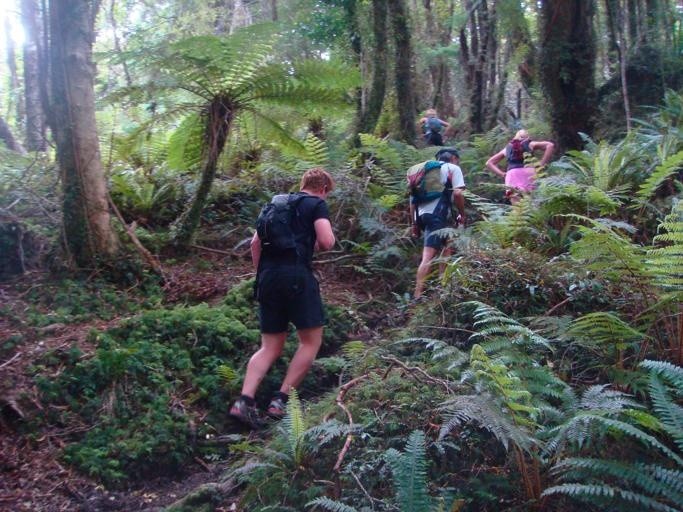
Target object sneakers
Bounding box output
[264,397,286,420]
[228,399,261,429]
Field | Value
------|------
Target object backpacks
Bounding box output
[428,117,441,133]
[509,139,528,162]
[256,195,318,256]
[406,161,445,201]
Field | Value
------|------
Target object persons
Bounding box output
[409,149,466,303]
[420,108,453,146]
[229,168,335,427]
[486,128,555,206]
